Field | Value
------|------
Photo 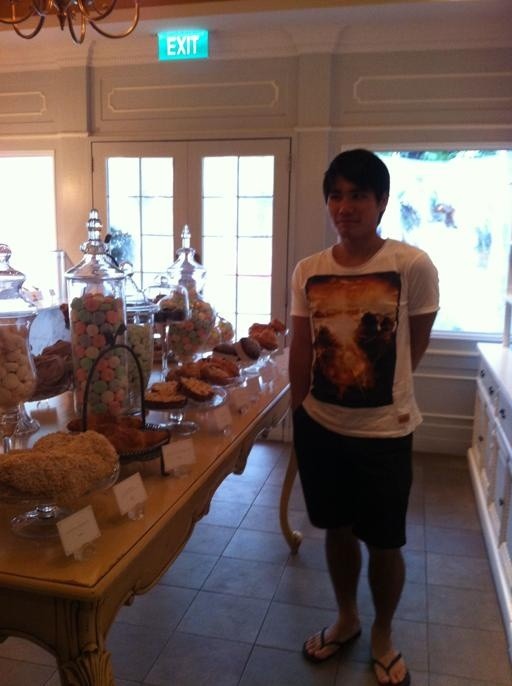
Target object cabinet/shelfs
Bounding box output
[409,334,479,456]
[469,340,512,654]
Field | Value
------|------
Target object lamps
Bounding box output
[0,0,140,46]
[157,28,209,61]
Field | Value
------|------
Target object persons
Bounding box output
[288,148,440,684]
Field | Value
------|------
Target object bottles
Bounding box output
[65,209,130,418]
[119,263,157,416]
[165,225,207,298]
[0,244,40,435]
[141,278,186,391]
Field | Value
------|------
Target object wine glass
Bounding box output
[0,458,120,545]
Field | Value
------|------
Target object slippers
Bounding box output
[371,651,411,686]
[303,626,361,665]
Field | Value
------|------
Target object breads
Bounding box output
[66,416,168,450]
[0,429,120,507]
[32,339,73,401]
[145,318,289,409]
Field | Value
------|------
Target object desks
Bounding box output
[1,348,308,686]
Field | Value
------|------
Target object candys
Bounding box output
[160,284,234,360]
[0,325,37,409]
[71,288,153,416]
